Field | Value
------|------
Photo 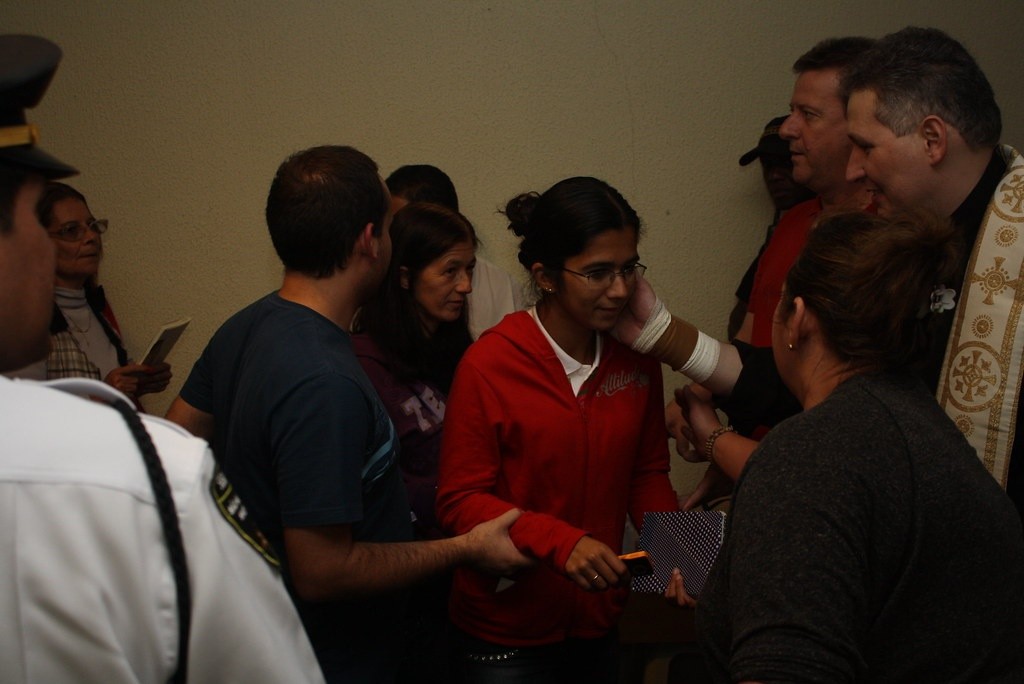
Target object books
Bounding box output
[631,510,726,594]
[138,315,190,365]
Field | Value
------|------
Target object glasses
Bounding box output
[553,260,648,293]
[47,217,109,242]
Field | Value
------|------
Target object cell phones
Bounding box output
[616,550,654,576]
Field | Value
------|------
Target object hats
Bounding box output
[0,33,81,188]
[739,113,793,166]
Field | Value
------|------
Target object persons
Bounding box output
[666,35,907,477]
[348,164,479,607]
[616,25,1023,513]
[0,33,327,684]
[434,175,704,683]
[162,147,537,684]
[671,209,1024,684]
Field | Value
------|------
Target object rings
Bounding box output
[592,574,599,582]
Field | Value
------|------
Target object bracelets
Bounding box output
[705,425,738,466]
